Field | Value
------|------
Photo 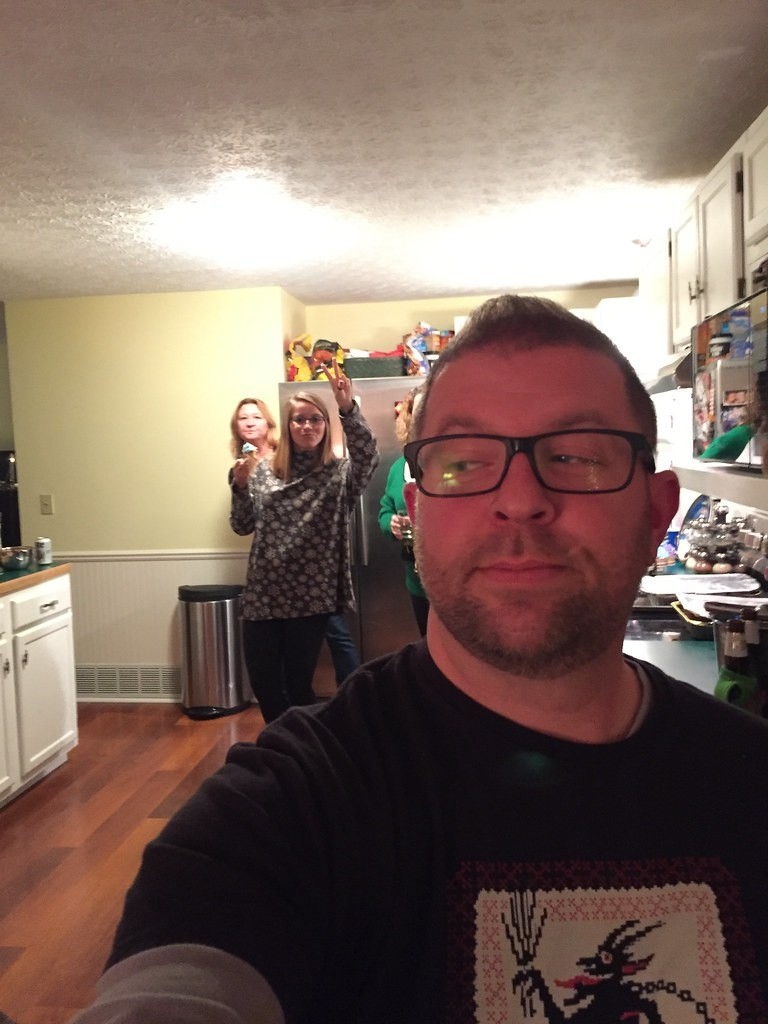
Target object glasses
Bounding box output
[291,415,327,426]
[403,426,659,496]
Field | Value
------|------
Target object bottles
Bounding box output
[723,605,763,679]
[708,333,735,358]
[423,350,440,370]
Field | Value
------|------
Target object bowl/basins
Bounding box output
[0,545,33,570]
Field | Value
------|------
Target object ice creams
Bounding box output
[242,443,257,459]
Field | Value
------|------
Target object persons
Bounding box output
[377,386,431,638]
[229,356,380,722]
[67,293,768,1024]
[229,398,362,690]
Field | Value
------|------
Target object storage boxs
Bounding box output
[344,355,404,377]
[427,330,455,354]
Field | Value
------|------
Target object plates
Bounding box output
[639,571,759,596]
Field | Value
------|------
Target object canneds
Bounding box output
[35,536,53,564]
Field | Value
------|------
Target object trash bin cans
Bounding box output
[177,586,251,721]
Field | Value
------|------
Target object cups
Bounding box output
[396,510,414,540]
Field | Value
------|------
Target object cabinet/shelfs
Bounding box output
[661,104,768,353]
[0,561,81,809]
